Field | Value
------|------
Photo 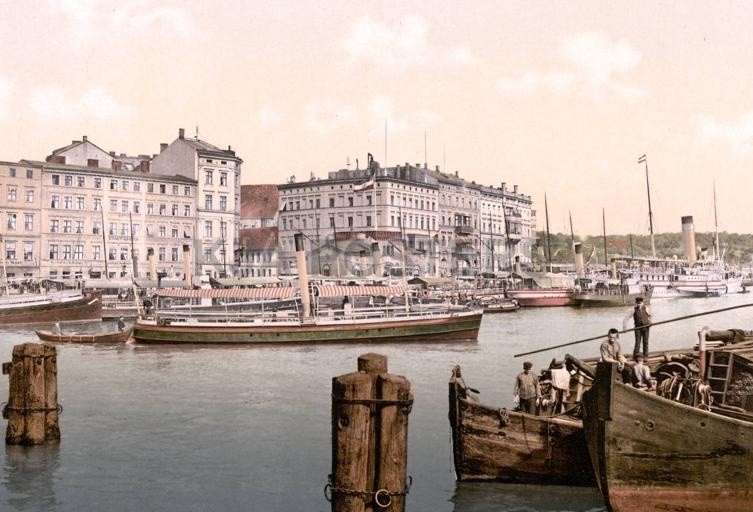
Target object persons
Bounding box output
[633,352,653,391]
[19,279,41,294]
[342,296,350,315]
[599,328,634,384]
[118,316,125,332]
[623,297,652,359]
[513,362,542,415]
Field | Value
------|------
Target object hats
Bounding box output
[635,297,644,303]
[523,362,533,371]
[634,353,644,358]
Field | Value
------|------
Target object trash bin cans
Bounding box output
[597,283,605,295]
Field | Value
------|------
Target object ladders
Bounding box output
[705,350,734,404]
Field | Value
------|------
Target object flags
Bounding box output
[638,154,646,163]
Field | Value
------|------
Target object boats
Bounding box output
[603,259,745,299]
[580,328,753,510]
[449,346,702,483]
[134,232,485,345]
[1,274,103,327]
[37,325,131,344]
[170,263,652,345]
